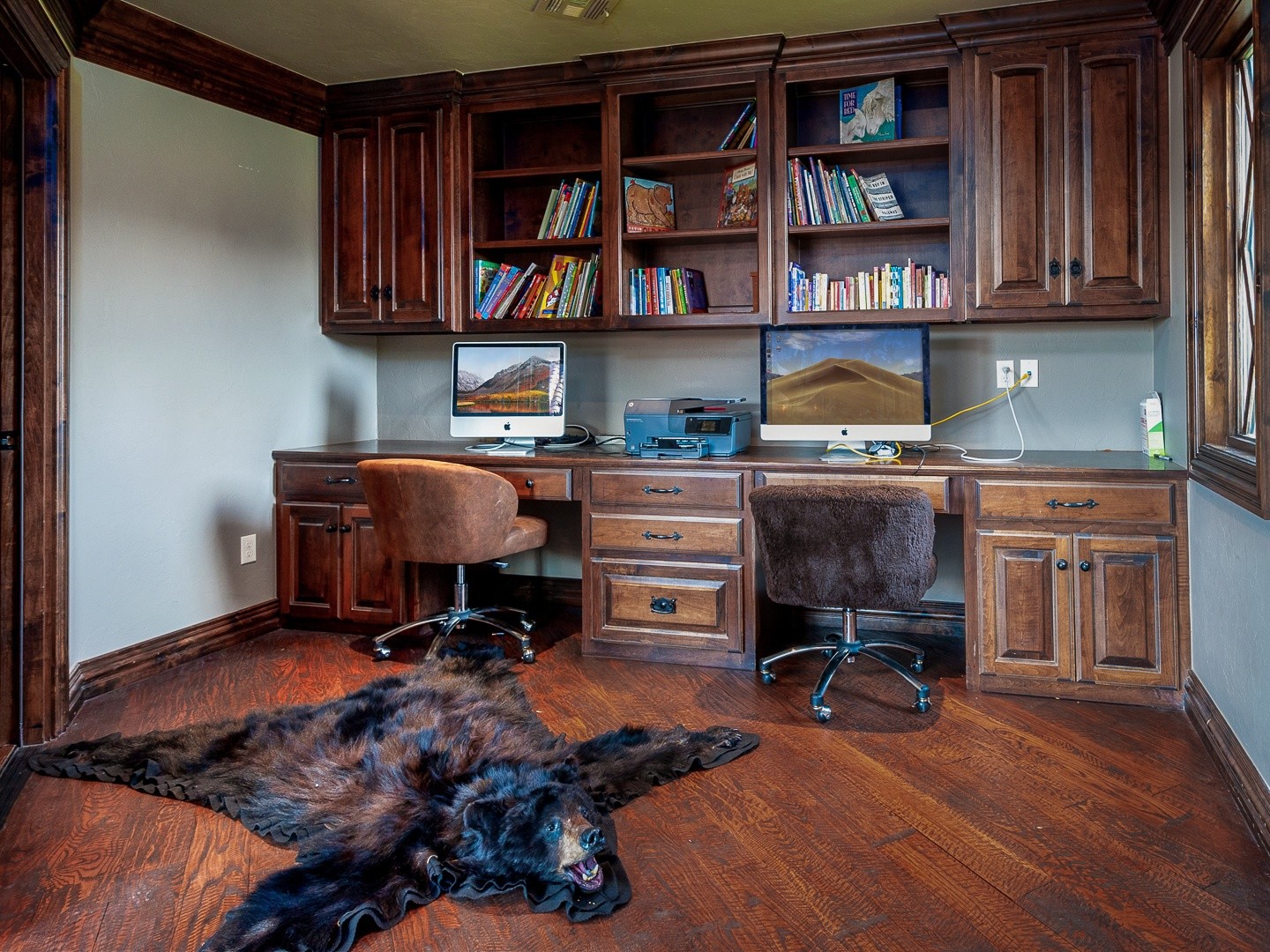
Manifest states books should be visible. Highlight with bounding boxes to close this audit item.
[472,76,952,320]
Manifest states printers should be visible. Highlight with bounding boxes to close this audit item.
[624,396,751,459]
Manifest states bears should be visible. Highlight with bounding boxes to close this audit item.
[7,624,761,952]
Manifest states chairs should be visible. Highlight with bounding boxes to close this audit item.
[748,484,941,723]
[351,456,551,660]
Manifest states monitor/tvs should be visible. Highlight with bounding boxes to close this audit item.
[760,323,932,441]
[450,341,565,438]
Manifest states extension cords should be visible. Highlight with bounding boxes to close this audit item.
[594,434,626,444]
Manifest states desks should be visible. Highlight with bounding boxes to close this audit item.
[270,439,1193,710]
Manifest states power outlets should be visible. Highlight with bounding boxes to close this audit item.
[996,359,1015,388]
[241,533,256,564]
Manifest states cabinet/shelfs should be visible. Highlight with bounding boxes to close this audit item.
[319,1,1172,335]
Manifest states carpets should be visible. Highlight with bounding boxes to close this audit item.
[28,638,761,952]
